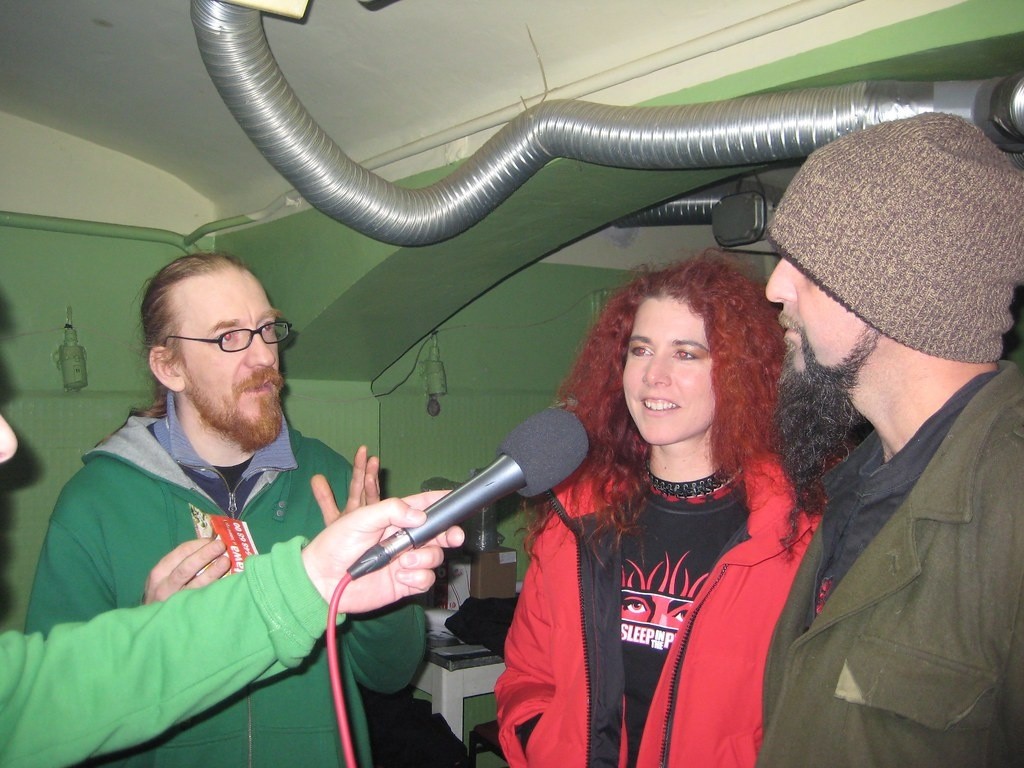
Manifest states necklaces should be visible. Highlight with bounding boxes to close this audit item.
[645,446,736,498]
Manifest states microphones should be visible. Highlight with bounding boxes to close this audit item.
[345,409,591,579]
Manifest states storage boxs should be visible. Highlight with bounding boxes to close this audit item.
[415,547,517,610]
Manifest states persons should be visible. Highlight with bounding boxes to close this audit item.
[755,114,1024,768]
[25,252,426,768]
[493,251,838,768]
[0,415,465,768]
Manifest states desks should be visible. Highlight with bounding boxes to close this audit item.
[409,645,510,768]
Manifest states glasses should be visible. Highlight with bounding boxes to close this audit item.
[162,317,293,352]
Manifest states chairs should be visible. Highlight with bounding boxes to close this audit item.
[469,720,508,768]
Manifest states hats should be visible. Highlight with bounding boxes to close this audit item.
[766,111,1024,359]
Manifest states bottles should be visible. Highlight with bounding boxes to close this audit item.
[467,469,497,551]
[61,331,88,389]
